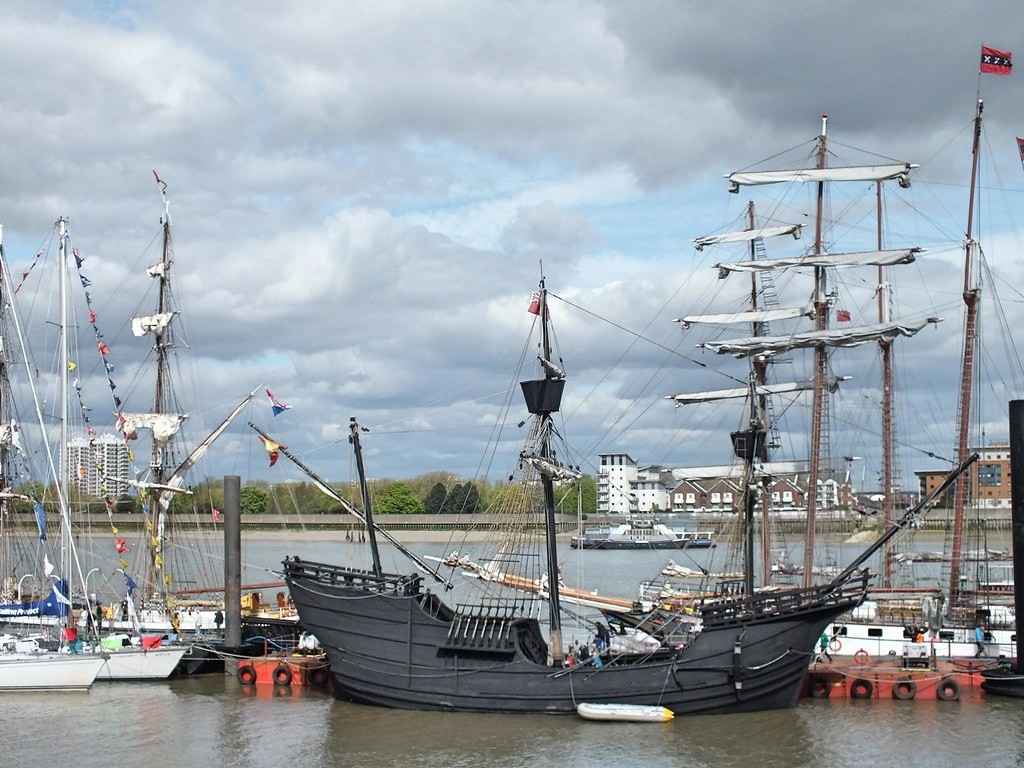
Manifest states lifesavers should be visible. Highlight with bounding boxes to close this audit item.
[851,678,873,699]
[237,665,256,685]
[854,650,869,665]
[937,678,961,701]
[308,667,329,686]
[809,676,831,699]
[829,640,842,651]
[894,678,916,700]
[273,666,292,686]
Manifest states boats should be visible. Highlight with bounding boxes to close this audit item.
[571,517,716,548]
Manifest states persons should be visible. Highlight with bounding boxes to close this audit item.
[194,610,203,640]
[562,621,610,669]
[974,625,985,659]
[214,606,223,637]
[820,633,833,665]
[63,624,77,656]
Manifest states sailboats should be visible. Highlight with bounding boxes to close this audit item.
[0,218,225,680]
[247,261,980,720]
[807,97,1023,699]
[0,169,110,693]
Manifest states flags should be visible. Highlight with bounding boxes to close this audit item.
[980,44,1012,75]
[5,234,288,625]
[836,310,850,322]
[527,289,551,322]
[1017,136,1024,163]
